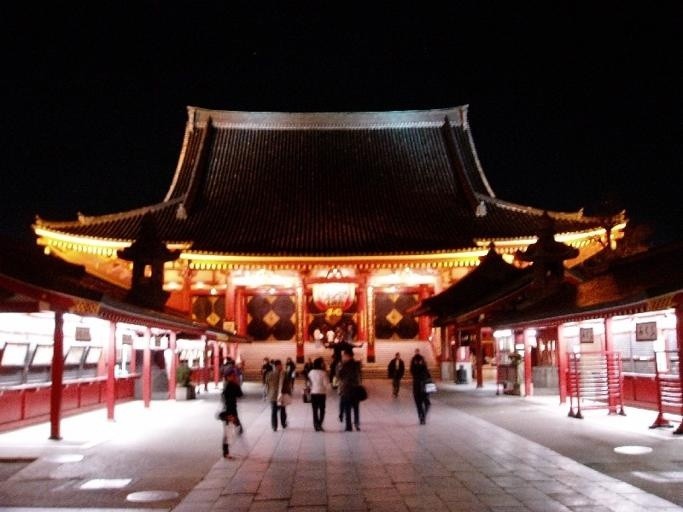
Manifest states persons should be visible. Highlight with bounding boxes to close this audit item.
[217,365,249,460]
[179,361,193,386]
[219,353,345,394]
[409,348,426,378]
[334,349,347,423]
[454,364,466,384]
[218,410,240,460]
[176,359,196,400]
[387,351,405,397]
[307,356,331,432]
[263,360,290,431]
[412,356,433,424]
[337,349,364,431]
[307,320,363,354]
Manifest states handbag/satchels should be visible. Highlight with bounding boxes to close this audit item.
[303,393,311,403]
[425,383,438,393]
[278,394,291,407]
[354,383,367,402]
